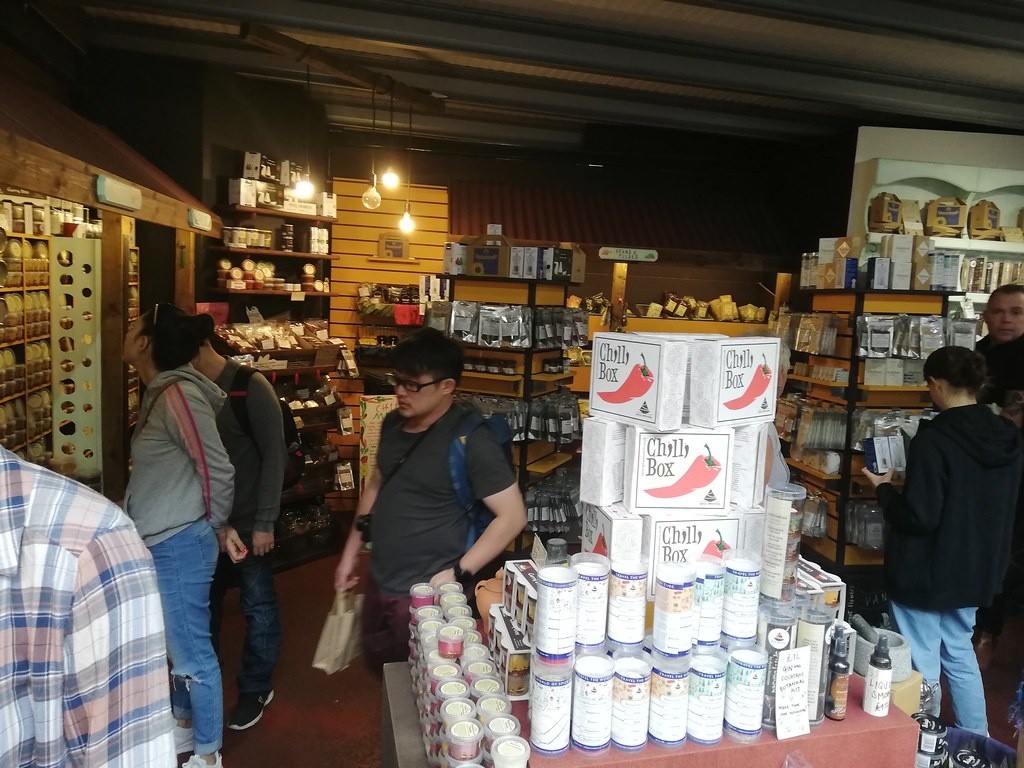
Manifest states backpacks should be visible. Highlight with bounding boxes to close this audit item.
[449,413,513,579]
[230,367,305,491]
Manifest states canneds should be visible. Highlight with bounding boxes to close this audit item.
[222,226,273,251]
[281,223,293,252]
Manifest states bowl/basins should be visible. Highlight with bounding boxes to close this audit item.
[0,198,53,465]
[216,260,325,292]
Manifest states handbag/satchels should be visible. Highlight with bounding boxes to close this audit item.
[312,578,368,676]
[355,515,373,542]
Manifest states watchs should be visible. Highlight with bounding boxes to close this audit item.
[453,563,471,585]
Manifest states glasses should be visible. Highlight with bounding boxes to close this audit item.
[384,371,443,392]
[154,303,171,325]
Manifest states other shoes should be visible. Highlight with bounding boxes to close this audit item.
[974,632,994,670]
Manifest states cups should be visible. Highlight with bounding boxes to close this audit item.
[547,538,568,563]
[408,569,537,768]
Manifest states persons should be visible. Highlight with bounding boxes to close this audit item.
[120,304,301,768]
[0,443,177,768]
[860,344,1024,741]
[973,284,1024,429]
[334,325,529,674]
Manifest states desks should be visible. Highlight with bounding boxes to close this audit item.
[379,621,920,768]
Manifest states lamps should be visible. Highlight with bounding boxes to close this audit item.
[380,79,401,189]
[400,98,415,233]
[294,67,314,198]
[363,85,380,210]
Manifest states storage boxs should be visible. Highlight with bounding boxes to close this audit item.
[926,197,967,227]
[871,192,903,223]
[419,223,572,301]
[666,294,688,316]
[867,235,960,293]
[970,199,1001,229]
[581,332,782,569]
[229,151,337,218]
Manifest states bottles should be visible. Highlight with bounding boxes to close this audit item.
[543,358,563,374]
[455,258,463,274]
[822,625,893,721]
[553,261,563,274]
[83,207,103,239]
[50,203,87,239]
[526,252,535,275]
[862,437,878,473]
[768,300,791,343]
[513,253,521,275]
[800,252,819,289]
[462,357,517,376]
[223,226,273,251]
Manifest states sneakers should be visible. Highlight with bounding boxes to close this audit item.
[182,750,223,768]
[171,715,194,754]
[227,687,275,729]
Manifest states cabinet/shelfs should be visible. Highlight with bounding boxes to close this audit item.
[0,232,140,460]
[776,273,966,623]
[356,305,432,381]
[218,206,339,371]
[442,273,585,558]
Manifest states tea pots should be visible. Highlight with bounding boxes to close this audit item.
[474,567,505,633]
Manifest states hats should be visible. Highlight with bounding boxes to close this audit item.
[174,308,214,339]
